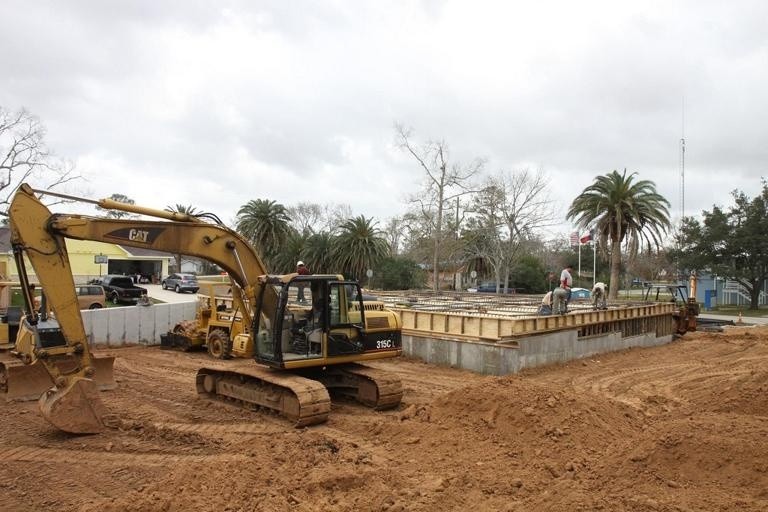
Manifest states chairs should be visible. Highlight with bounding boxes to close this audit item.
[299,299,332,353]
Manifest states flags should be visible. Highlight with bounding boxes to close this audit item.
[571,231,579,246]
[581,229,593,244]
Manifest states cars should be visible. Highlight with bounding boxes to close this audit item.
[161,271,200,294]
[414,260,459,273]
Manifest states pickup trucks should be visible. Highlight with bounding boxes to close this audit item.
[98,274,148,306]
[476,281,516,294]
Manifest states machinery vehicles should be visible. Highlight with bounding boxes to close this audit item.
[159,275,256,357]
[8,173,407,443]
[638,271,704,337]
[0,260,118,402]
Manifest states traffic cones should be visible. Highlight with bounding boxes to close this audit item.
[735,310,744,323]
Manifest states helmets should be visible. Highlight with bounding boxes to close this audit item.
[297,260,305,267]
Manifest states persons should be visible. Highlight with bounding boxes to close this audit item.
[590,282,609,311]
[538,265,573,316]
[294,259,309,302]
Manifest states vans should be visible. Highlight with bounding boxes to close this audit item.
[30,283,106,314]
